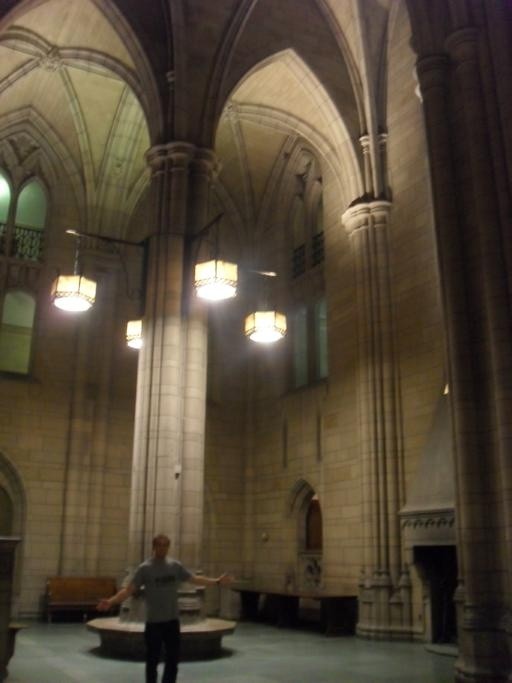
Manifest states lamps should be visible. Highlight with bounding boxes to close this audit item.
[49,211,289,350]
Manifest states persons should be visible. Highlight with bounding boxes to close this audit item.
[96,534,236,683]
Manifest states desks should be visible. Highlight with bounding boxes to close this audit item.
[230,583,359,638]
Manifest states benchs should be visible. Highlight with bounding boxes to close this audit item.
[44,577,120,624]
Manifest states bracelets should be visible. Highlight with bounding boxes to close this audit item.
[217,577,219,584]
